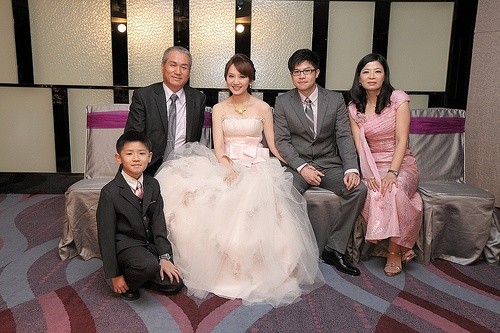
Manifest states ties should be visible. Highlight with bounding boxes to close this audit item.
[136,181,143,199]
[163,94,179,164]
[304,97,315,139]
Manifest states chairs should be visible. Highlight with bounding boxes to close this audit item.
[58,103,131,263]
[275,93,363,264]
[199,107,212,150]
[408,109,500,266]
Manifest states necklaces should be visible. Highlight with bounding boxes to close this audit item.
[231,93,251,116]
[366,100,376,107]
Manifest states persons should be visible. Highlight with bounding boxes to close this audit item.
[95,130,184,300]
[152,52,326,308]
[346,53,424,276]
[273,48,368,276]
[123,46,212,178]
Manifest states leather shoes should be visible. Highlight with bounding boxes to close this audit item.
[322,246,361,276]
[121,286,139,300]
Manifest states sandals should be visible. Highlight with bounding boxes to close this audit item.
[384,249,417,276]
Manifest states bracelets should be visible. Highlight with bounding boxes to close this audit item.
[388,169,399,178]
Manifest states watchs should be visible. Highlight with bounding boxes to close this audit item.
[159,253,171,260]
